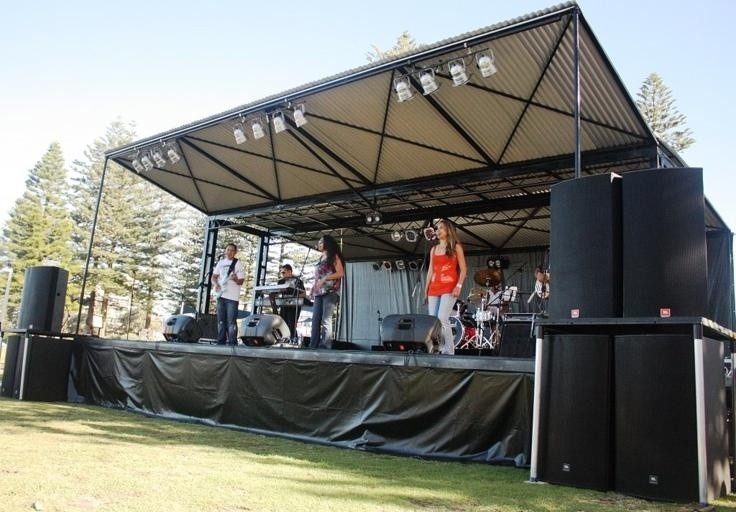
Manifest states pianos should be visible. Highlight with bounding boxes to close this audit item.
[254,297,313,307]
[254,283,304,295]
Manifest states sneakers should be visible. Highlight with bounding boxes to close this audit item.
[439,346,455,356]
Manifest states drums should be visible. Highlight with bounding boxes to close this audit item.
[467,287,486,304]
[475,310,492,322]
[449,315,478,348]
[452,299,469,315]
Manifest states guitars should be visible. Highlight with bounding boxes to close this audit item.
[212,268,240,299]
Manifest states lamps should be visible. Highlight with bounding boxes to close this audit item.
[231,98,308,145]
[391,49,498,104]
[128,141,181,175]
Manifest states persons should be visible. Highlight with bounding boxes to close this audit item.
[486,283,506,336]
[277,264,307,346]
[210,242,245,348]
[534,268,551,313]
[421,219,467,357]
[308,235,346,352]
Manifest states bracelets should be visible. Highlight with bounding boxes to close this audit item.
[456,283,463,288]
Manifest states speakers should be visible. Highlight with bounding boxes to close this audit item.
[16,266,68,333]
[0,336,73,402]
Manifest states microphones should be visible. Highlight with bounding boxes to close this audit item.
[310,244,317,249]
[217,252,227,258]
[430,231,440,241]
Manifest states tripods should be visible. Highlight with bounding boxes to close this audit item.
[459,280,502,349]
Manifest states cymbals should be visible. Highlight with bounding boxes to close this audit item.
[474,269,502,287]
